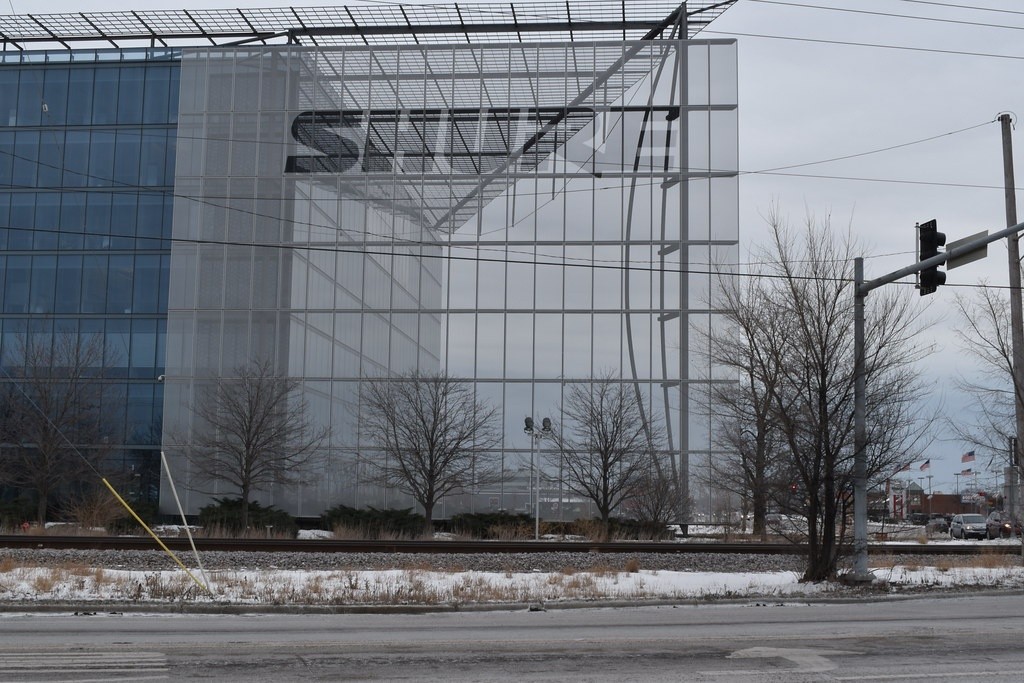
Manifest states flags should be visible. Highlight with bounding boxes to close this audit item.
[920,459,930,471]
[961,451,975,463]
[961,469,971,476]
[893,463,910,474]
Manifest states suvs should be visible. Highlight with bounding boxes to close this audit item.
[949,513,988,540]
[986,510,1024,540]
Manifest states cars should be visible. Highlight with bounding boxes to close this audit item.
[699,512,823,525]
[912,512,954,526]
[926,520,949,535]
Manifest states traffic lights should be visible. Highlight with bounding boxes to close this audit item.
[792,484,797,493]
[919,219,947,297]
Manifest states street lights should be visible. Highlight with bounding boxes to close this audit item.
[926,475,933,521]
[907,479,913,519]
[524,417,552,540]
[972,472,981,512]
[991,469,1002,510]
[918,477,924,513]
[954,473,962,514]
